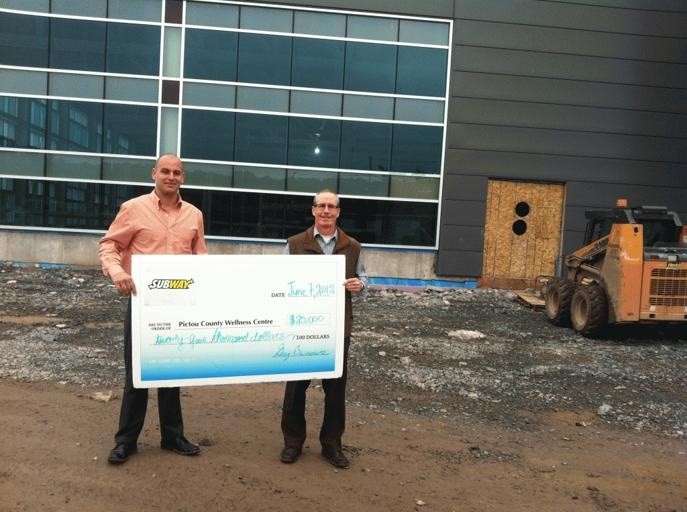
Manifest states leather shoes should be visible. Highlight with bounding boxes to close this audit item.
[108,445,137,463]
[160,438,201,455]
[281,445,301,462]
[322,446,349,468]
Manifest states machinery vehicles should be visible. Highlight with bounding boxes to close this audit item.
[517,194,686,343]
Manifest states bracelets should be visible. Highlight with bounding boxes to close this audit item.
[361,282,364,290]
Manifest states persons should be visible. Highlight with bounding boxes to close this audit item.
[96,153,207,461]
[279,188,367,467]
